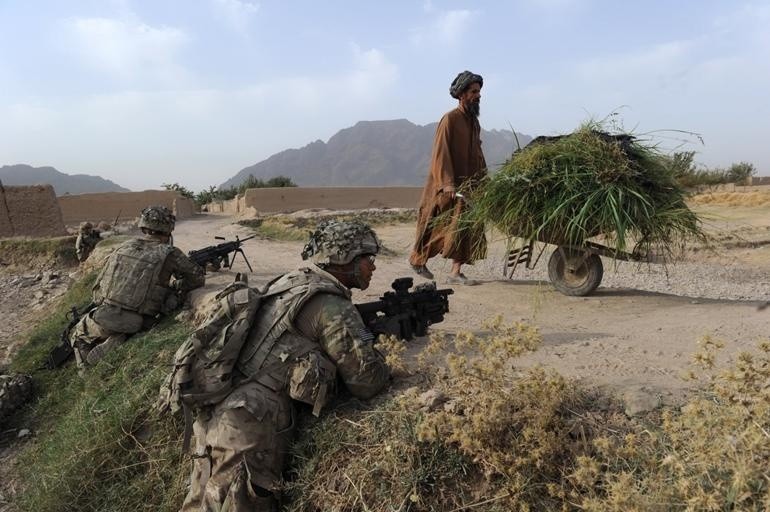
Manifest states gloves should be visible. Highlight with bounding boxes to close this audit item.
[414,281,436,293]
[372,312,409,341]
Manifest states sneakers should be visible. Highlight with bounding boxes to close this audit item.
[446,272,476,287]
[410,259,434,279]
[86,331,127,365]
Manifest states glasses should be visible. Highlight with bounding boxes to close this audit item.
[365,255,376,264]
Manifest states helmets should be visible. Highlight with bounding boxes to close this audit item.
[80,221,92,231]
[303,221,381,266]
[137,205,175,234]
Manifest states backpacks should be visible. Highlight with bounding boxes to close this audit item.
[0,371,33,418]
[153,272,335,453]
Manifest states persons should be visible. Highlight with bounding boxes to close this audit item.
[74,221,103,262]
[172,217,401,512]
[68,205,206,380]
[408,70,488,286]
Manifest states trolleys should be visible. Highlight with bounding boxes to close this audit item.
[455,190,695,298]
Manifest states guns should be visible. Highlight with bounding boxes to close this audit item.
[188,237,255,272]
[354,277,454,341]
